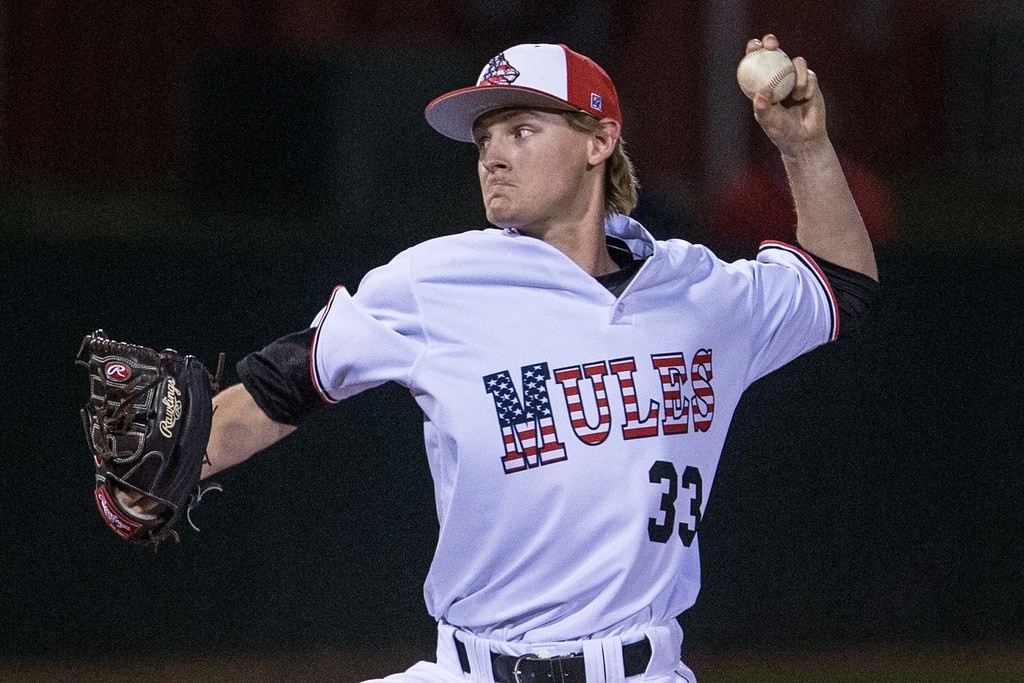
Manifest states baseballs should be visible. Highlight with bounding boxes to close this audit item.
[736,47,798,104]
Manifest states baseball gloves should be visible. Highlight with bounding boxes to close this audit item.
[76,327,214,548]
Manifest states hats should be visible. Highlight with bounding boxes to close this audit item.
[425,44,622,144]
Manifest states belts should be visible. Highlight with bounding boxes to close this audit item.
[453,630,651,683]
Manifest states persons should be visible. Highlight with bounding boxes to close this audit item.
[73,34,887,683]
